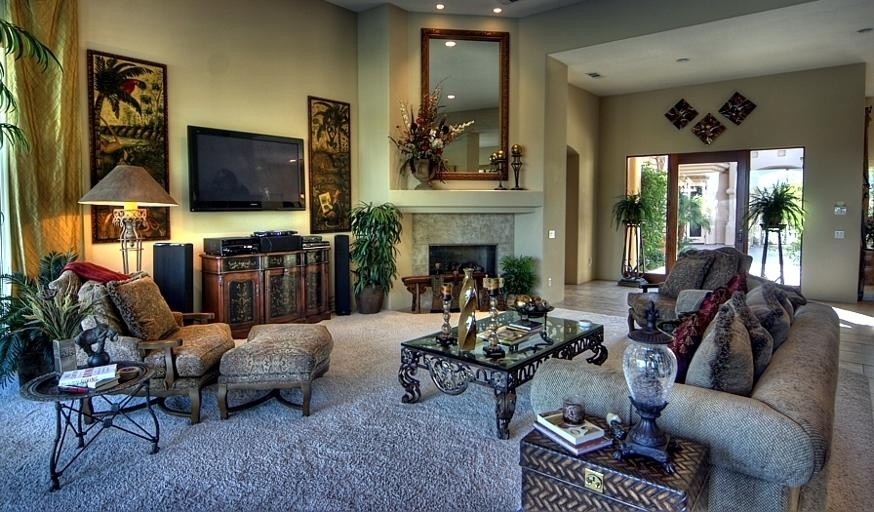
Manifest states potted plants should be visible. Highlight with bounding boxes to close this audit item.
[609,192,654,232]
[737,183,809,241]
[0,247,122,390]
[347,200,404,314]
[499,255,540,311]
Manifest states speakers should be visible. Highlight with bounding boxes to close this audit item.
[153,242,194,325]
[334,235,350,316]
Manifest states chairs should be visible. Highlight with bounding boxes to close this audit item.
[627,248,752,333]
[50,270,234,424]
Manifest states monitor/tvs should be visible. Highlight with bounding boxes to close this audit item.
[187,125,306,212]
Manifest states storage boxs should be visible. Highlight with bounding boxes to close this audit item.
[519,415,711,512]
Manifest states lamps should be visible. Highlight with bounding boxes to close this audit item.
[77,164,180,276]
[612,300,683,473]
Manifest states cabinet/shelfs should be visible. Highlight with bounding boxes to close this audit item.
[199,247,331,340]
[760,224,786,284]
[617,224,648,288]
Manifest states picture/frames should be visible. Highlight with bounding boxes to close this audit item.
[86,48,171,244]
[307,95,352,233]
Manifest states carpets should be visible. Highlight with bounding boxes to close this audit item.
[0,309,873,512]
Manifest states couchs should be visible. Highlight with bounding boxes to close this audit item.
[530,301,840,512]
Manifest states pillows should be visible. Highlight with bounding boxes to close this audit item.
[661,250,794,395]
[106,273,179,342]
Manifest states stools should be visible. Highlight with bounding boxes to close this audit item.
[218,323,333,420]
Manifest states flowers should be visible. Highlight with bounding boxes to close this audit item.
[389,84,474,184]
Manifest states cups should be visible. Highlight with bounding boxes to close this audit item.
[561,394,584,425]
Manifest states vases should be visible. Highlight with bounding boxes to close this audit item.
[410,159,437,190]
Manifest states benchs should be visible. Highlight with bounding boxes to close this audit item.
[402,274,486,312]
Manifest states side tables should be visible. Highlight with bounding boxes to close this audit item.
[20,361,160,492]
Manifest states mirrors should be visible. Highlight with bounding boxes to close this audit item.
[420,28,510,180]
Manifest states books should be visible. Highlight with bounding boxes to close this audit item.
[476,319,544,345]
[533,409,613,456]
[57,364,120,393]
